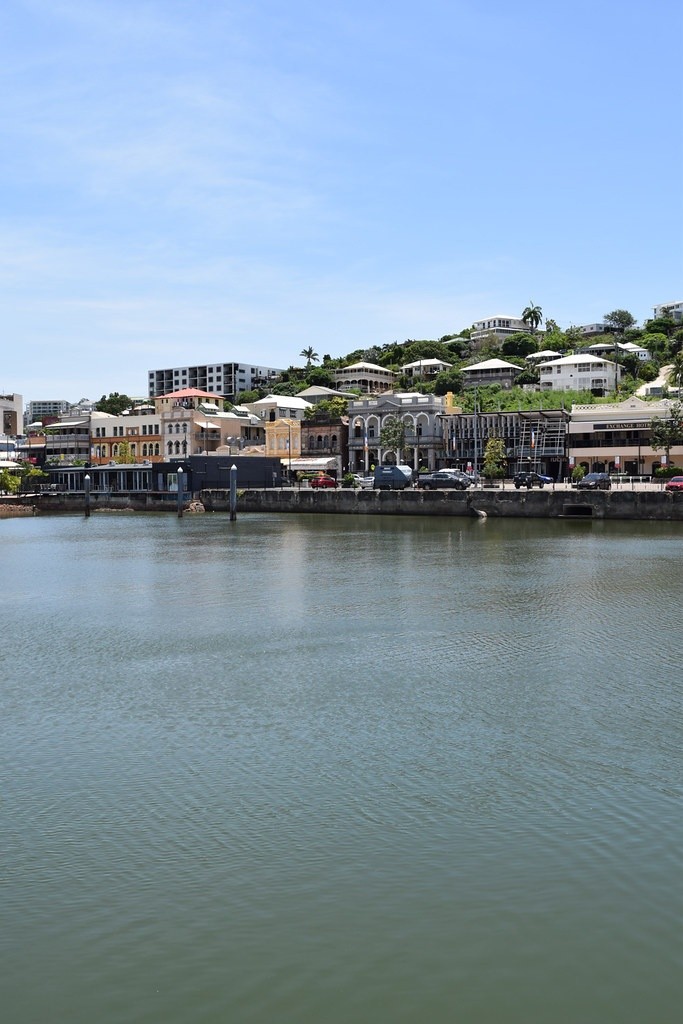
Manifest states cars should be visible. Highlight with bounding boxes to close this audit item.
[513,472,554,489]
[310,477,338,489]
[666,476,683,491]
[360,477,375,489]
[417,468,480,491]
[578,472,611,490]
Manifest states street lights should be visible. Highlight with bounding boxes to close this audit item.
[468,379,482,488]
[283,423,291,488]
[614,331,618,393]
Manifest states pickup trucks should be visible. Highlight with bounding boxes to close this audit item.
[332,474,364,483]
